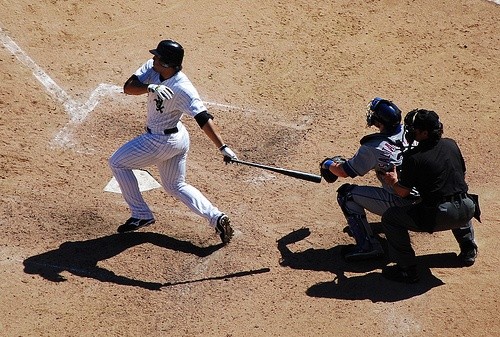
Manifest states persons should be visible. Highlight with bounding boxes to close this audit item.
[381,108,478,281]
[320,98,418,258]
[108,39,240,246]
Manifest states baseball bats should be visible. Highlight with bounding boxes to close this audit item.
[223,155,322,184]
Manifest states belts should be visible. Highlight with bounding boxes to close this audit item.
[146,126,178,135]
[403,195,419,201]
[446,193,465,202]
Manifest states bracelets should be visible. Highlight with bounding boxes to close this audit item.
[219,144,228,151]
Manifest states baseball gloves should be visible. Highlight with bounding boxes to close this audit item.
[319,155,347,184]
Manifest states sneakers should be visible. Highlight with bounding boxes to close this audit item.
[383,263,420,284]
[116,217,156,233]
[457,251,475,266]
[215,214,235,243]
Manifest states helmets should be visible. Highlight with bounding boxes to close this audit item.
[149,40,185,68]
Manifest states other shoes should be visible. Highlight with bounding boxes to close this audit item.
[340,243,384,262]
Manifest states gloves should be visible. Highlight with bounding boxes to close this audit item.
[147,83,175,101]
[219,144,239,165]
